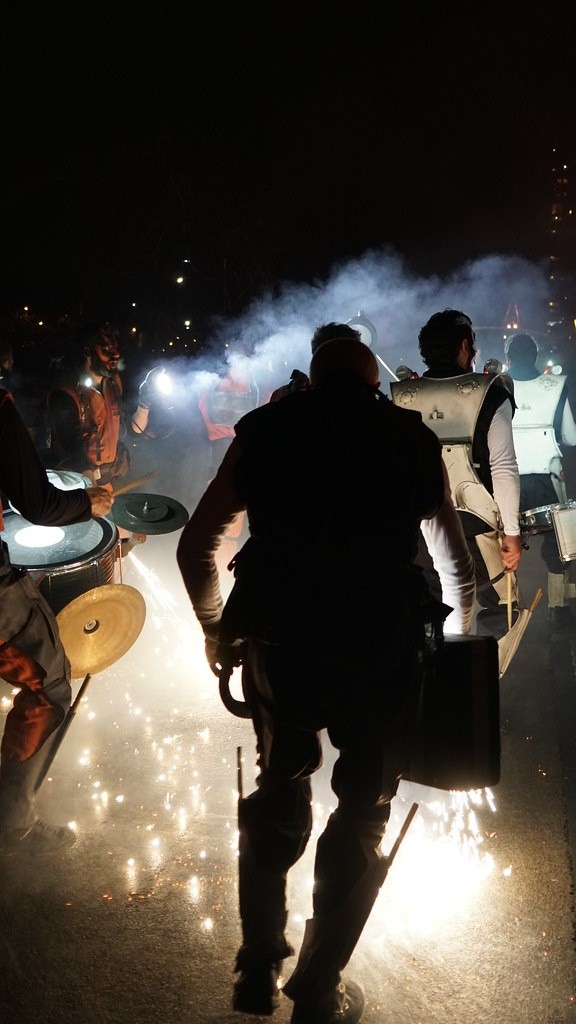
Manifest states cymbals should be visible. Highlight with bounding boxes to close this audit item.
[52,582,148,682]
[105,491,190,537]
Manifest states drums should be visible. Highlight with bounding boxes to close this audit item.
[522,500,576,537]
[0,507,120,616]
[43,466,94,492]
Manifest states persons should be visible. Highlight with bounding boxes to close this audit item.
[176,322,477,1024]
[0,302,576,856]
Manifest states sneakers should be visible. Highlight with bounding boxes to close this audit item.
[0,820,75,856]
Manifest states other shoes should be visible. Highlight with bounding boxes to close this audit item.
[289,981,364,1024]
[232,964,282,1015]
[551,606,576,627]
